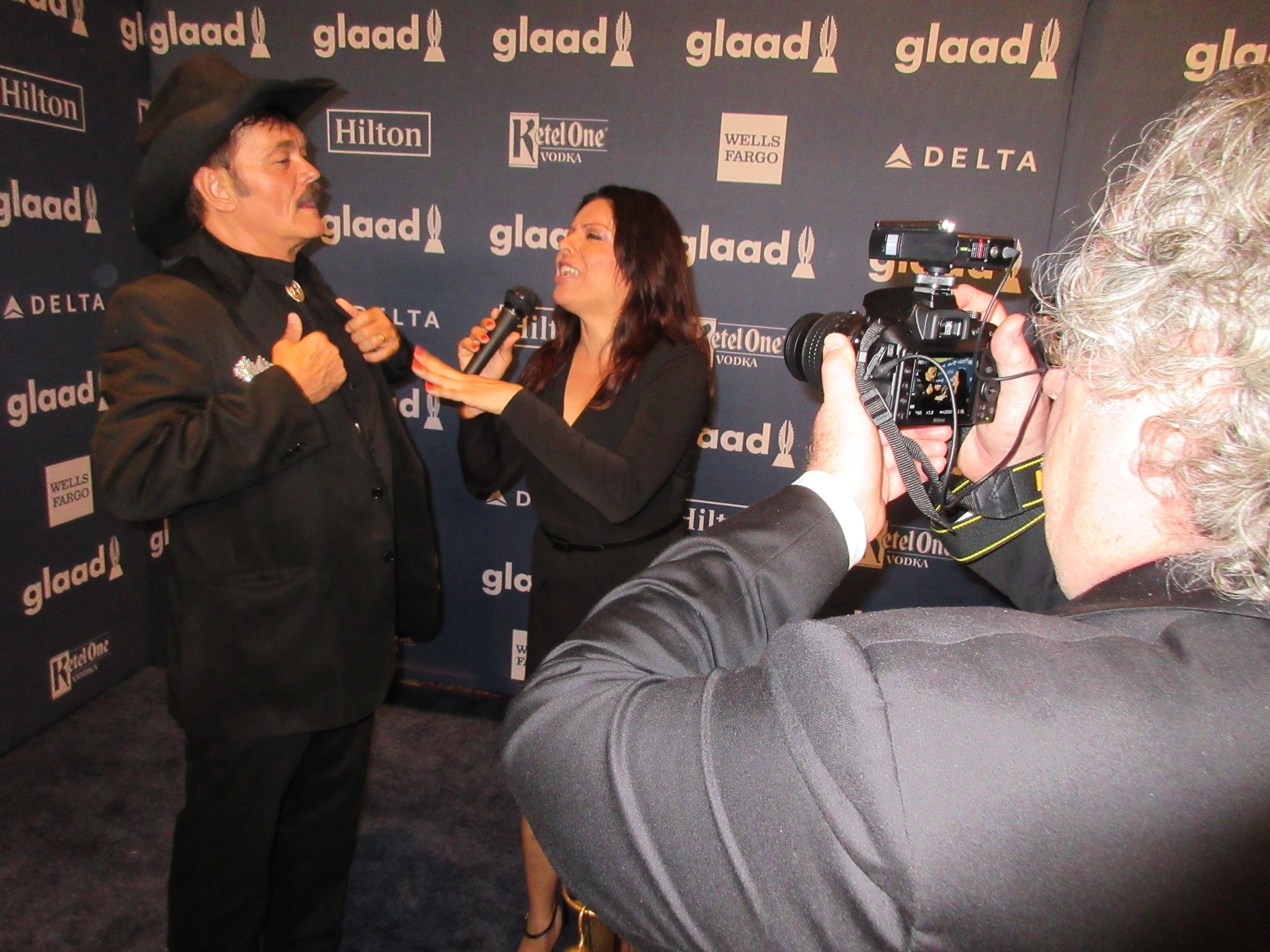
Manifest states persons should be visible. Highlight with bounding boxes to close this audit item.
[496,60,1270,952]
[87,49,451,952]
[410,180,719,952]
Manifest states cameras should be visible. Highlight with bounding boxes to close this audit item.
[782,220,1022,425]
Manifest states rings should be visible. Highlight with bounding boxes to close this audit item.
[378,334,386,345]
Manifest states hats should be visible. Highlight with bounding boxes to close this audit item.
[126,49,337,260]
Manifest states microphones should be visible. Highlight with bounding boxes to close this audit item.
[461,286,539,376]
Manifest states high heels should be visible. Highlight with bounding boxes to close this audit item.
[523,904,572,952]
